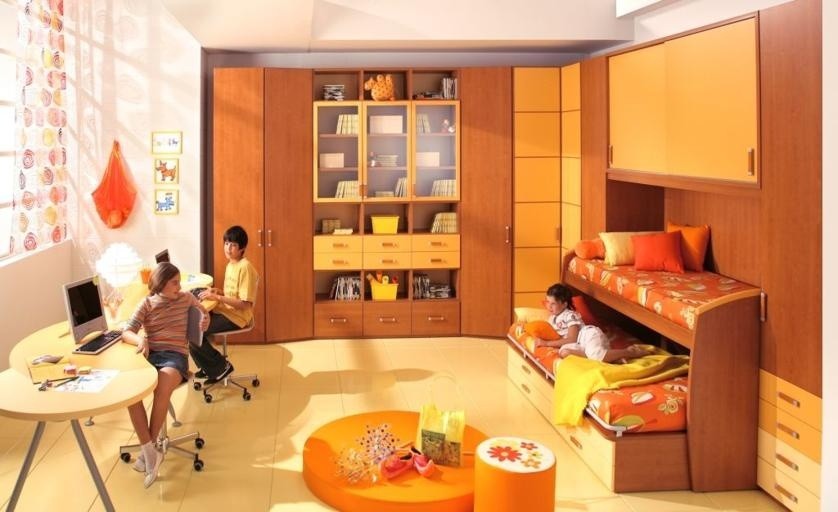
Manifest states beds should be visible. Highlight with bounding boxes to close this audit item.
[507,245,762,495]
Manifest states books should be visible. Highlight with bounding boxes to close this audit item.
[26,353,79,385]
[319,74,461,302]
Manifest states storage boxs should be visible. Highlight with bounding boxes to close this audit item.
[413,150,440,169]
[367,114,404,133]
[318,153,349,168]
[371,214,400,234]
[371,280,398,300]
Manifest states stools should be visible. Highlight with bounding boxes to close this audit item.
[473,435,556,511]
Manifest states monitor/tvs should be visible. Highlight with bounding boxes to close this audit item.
[155,248,168,264]
[63,275,107,343]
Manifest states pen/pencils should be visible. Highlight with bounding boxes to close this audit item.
[55,376,79,388]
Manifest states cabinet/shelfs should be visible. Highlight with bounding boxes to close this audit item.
[211,66,315,349]
[603,11,761,199]
[456,65,560,345]
[754,2,822,512]
[560,57,664,269]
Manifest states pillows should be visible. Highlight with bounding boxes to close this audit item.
[576,224,712,274]
[514,295,608,342]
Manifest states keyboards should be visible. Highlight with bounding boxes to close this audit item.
[189,288,209,300]
[72,330,122,354]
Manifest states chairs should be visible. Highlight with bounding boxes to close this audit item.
[192,277,266,405]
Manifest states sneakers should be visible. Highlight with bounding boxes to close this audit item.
[203,361,233,386]
[194,367,210,379]
[380,453,413,478]
[412,446,436,476]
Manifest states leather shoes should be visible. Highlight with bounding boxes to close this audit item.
[134,456,147,473]
[144,452,164,487]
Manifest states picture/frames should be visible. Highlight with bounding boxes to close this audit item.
[153,157,179,186]
[153,187,180,217]
[149,131,184,156]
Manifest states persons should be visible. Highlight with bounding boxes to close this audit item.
[120,262,210,490]
[538,284,658,367]
[190,224,259,387]
